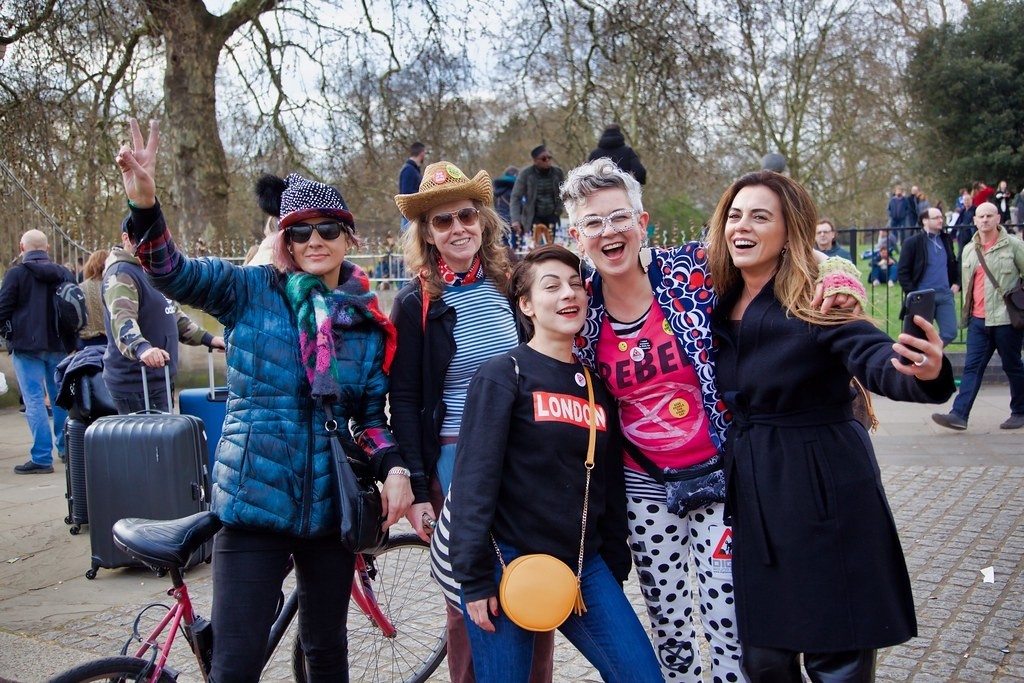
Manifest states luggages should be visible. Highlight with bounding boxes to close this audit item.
[62,346,230,580]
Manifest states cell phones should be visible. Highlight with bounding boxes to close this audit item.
[899,288,937,365]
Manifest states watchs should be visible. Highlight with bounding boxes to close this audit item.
[388,468,411,479]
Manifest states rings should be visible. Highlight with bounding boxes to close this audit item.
[914,354,926,367]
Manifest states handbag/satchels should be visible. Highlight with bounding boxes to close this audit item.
[499,554,587,632]
[1004,277,1024,330]
[329,429,389,554]
[849,376,878,437]
[662,453,728,515]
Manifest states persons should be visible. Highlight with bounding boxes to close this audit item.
[388,161,555,683]
[115,117,414,683]
[390,123,1024,683]
[0,173,287,475]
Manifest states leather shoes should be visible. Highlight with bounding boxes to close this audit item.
[61,456,65,463]
[13,460,54,474]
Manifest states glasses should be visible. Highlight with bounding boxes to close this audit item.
[535,156,552,162]
[814,230,834,237]
[575,209,638,239]
[284,221,343,243]
[927,216,945,221]
[426,207,480,233]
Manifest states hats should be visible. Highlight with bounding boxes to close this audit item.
[254,173,354,231]
[532,145,546,158]
[391,161,493,222]
[122,216,130,232]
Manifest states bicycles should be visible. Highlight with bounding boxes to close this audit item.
[40,509,449,683]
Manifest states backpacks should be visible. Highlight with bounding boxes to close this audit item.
[49,280,88,339]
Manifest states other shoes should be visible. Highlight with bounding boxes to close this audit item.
[873,279,880,287]
[1000,415,1024,429]
[931,412,967,430]
[888,281,894,287]
